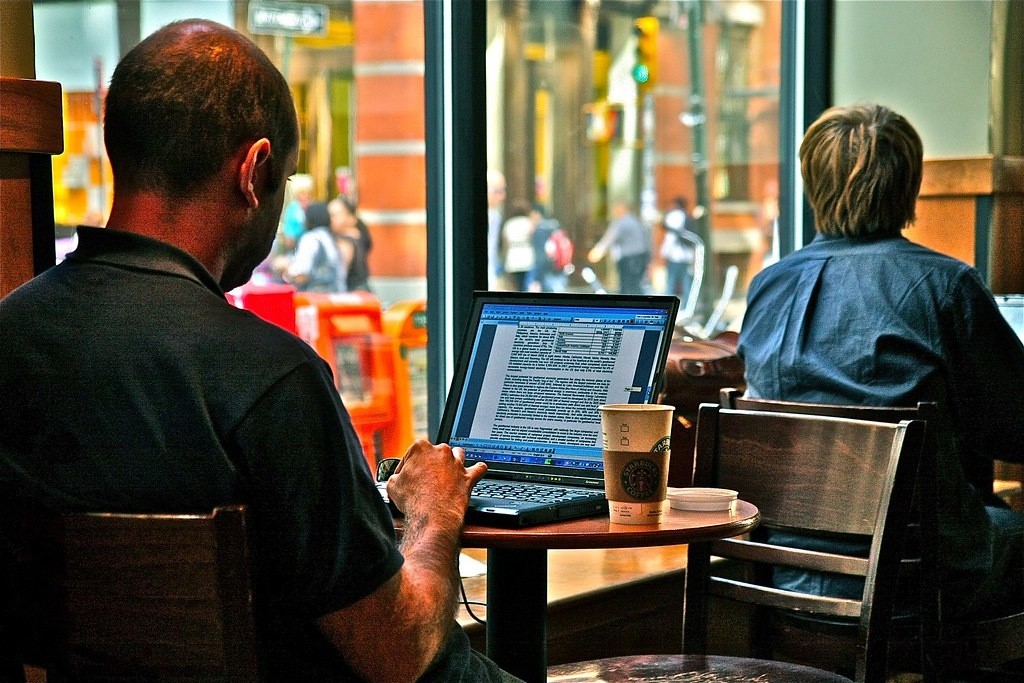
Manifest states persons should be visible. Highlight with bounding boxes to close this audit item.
[660,193,696,311]
[1,17,528,683]
[735,101,1024,683]
[269,173,372,295]
[587,197,651,295]
[500,197,573,293]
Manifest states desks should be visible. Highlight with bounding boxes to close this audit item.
[387,499,760,683]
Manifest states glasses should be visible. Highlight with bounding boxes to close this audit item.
[376,458,401,481]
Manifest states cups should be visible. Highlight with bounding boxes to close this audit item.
[598,403,675,525]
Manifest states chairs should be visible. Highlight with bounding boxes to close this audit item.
[0,502,257,683]
[680,403,929,683]
[718,385,1024,683]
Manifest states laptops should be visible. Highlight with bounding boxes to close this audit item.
[372,292,679,527]
